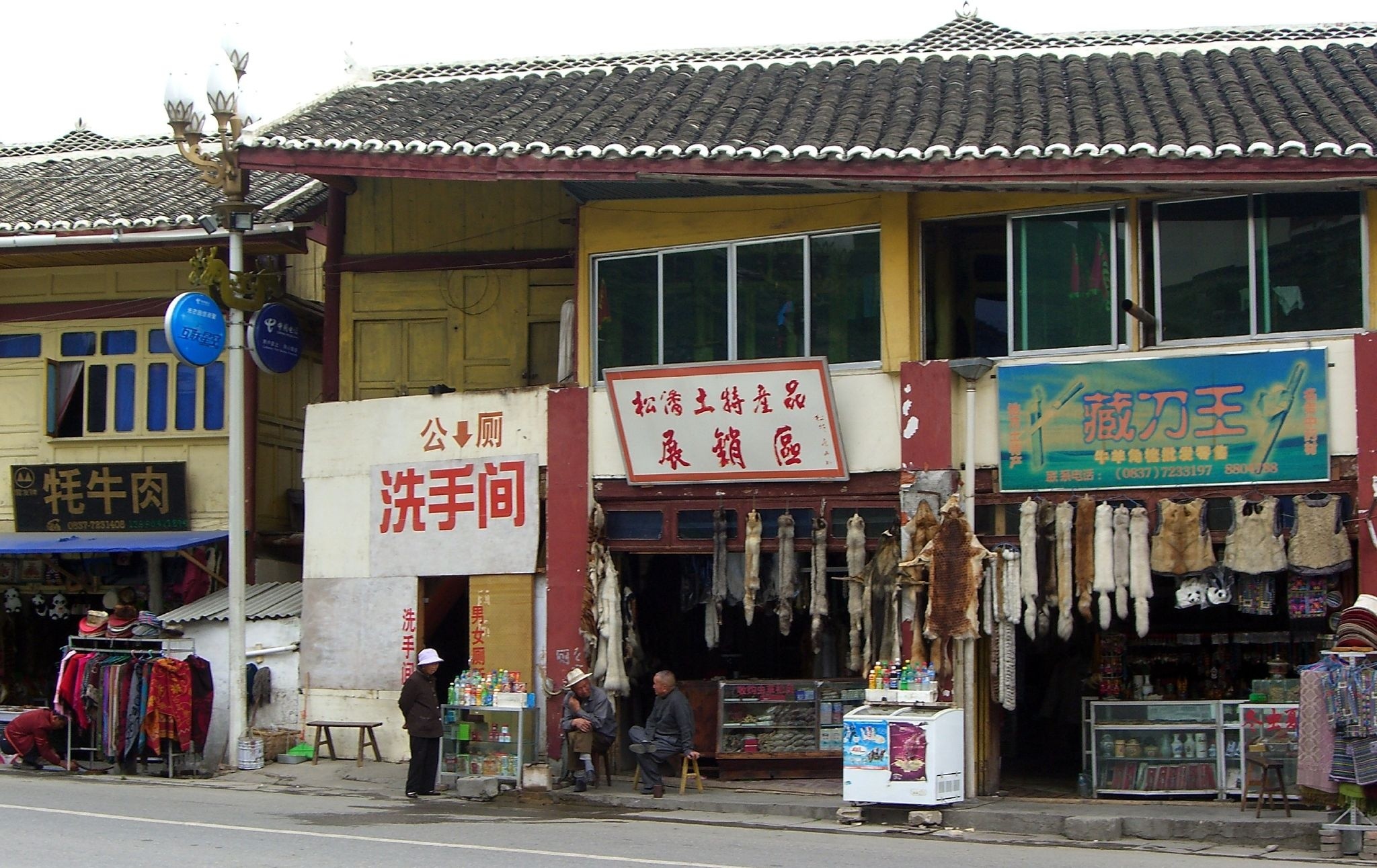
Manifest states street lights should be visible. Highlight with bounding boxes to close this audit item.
[161,27,309,768]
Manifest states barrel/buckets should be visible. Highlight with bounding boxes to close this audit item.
[238,726,265,770]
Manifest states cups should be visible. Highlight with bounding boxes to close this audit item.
[733,672,740,679]
[527,693,535,707]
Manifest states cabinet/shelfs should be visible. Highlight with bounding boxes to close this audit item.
[716,680,869,781]
[437,704,540,787]
[1081,696,1301,800]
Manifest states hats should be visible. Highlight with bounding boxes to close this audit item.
[564,668,593,687]
[76,604,163,639]
[1336,594,1377,649]
[417,649,444,665]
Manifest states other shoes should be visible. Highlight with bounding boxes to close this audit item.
[407,791,419,798]
[12,758,44,770]
[629,742,657,753]
[586,770,596,783]
[420,791,442,797]
[573,779,588,793]
[639,786,666,795]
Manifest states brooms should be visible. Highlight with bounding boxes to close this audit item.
[286,672,314,759]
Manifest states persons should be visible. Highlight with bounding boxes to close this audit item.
[0,708,80,770]
[560,668,618,786]
[629,670,700,798]
[398,648,444,798]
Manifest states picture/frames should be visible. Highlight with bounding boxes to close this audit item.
[0,557,45,583]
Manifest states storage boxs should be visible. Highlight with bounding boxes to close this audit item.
[493,692,536,708]
[0,705,49,721]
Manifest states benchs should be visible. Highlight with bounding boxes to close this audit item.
[633,753,703,795]
[306,721,383,767]
[1241,757,1291,818]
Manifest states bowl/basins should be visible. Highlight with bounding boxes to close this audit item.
[500,671,527,692]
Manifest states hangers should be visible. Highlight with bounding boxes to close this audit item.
[94,647,198,666]
[1169,485,1194,501]
[1302,488,1330,501]
[1295,654,1377,685]
[1018,489,1056,508]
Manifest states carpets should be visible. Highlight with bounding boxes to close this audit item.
[1067,488,1080,509]
[1242,481,1266,503]
[1101,494,1142,510]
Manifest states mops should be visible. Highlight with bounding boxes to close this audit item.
[217,663,258,771]
[250,666,271,726]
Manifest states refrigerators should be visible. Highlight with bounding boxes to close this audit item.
[842,704,966,805]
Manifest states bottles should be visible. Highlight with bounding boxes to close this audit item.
[869,658,935,691]
[446,668,509,706]
[1098,728,1218,759]
[1133,675,1144,699]
[1142,675,1153,695]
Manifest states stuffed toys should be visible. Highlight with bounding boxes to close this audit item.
[1,587,70,621]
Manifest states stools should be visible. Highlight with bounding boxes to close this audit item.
[592,748,612,788]
